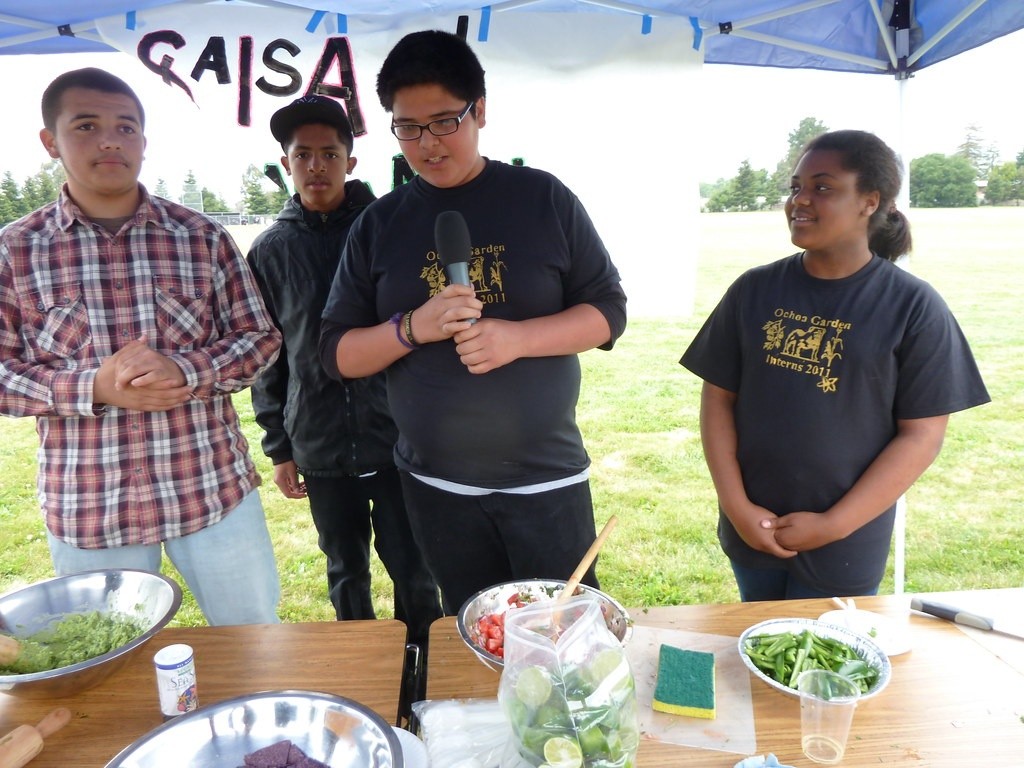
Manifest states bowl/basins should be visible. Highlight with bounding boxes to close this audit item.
[0,569,183,700]
[103,690,403,768]
[456,579,632,674]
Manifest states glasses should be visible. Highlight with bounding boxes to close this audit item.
[391,100,474,141]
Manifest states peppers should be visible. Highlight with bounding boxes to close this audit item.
[745,629,878,699]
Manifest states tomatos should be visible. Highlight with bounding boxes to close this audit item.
[482,594,531,660]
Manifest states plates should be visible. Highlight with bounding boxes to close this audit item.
[822,610,911,655]
[392,727,427,768]
[739,617,891,705]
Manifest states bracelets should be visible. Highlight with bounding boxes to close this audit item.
[405,310,418,345]
[389,312,420,349]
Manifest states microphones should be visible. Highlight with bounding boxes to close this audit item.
[436,210,477,329]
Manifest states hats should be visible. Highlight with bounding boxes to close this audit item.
[270,95,353,147]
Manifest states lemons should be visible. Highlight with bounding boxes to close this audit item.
[508,649,639,768]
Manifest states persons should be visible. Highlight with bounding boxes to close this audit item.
[315,30,628,617]
[678,130,991,603]
[247,95,442,654]
[0,66,282,629]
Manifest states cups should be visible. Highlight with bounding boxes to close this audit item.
[153,644,199,720]
[797,670,861,764]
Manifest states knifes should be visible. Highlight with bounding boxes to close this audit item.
[911,598,1024,637]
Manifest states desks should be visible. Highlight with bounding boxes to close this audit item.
[0,587,1024,767]
